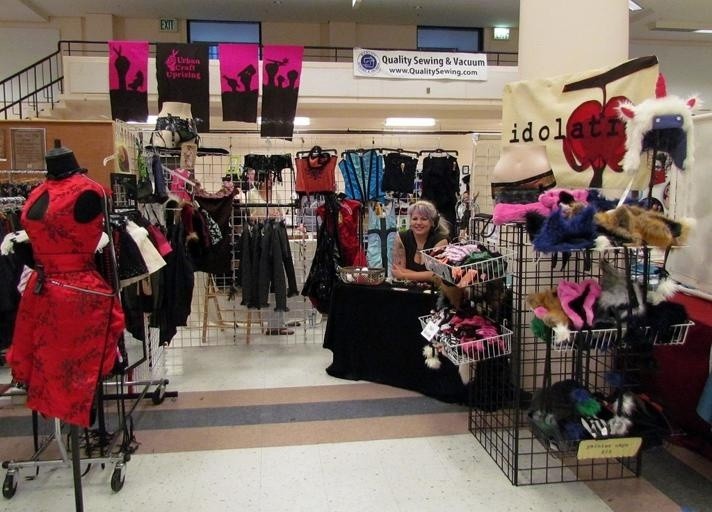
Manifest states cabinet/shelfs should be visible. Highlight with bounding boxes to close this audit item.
[465,213,695,486]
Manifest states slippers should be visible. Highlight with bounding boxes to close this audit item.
[266,327,295,335]
[288,321,300,326]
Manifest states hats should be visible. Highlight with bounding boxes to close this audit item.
[528,267,688,349]
[612,94,704,173]
[493,188,689,247]
[528,377,671,461]
[420,304,506,368]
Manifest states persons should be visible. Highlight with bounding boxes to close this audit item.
[490,79,558,203]
[391,200,449,283]
[7,146,126,428]
[233,171,286,222]
[152,102,198,149]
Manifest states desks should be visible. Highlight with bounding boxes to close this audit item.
[336,279,514,412]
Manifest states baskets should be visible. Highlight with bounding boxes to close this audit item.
[339,266,386,285]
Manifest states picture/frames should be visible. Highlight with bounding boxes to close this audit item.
[10,127,47,172]
